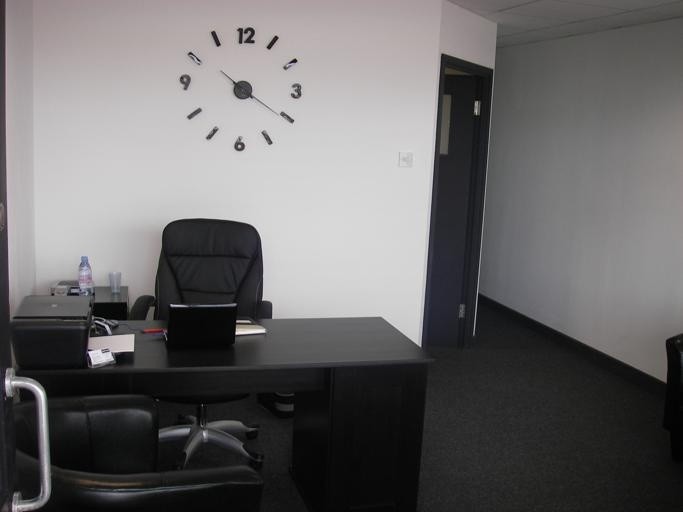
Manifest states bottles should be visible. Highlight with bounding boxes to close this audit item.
[76,256,93,296]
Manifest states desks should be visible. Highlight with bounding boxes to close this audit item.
[16,317,436,512]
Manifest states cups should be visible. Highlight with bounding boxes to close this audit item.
[108,272,121,294]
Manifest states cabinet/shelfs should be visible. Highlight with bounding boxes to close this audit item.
[52,286,128,320]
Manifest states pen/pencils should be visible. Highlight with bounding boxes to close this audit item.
[141,328,164,332]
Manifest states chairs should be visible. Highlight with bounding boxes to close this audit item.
[5,394,267,512]
[128,218,272,469]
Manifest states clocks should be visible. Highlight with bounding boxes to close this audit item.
[179,26,303,151]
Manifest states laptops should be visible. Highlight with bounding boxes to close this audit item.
[163,303,238,353]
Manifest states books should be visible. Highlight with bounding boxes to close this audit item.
[234,315,267,336]
